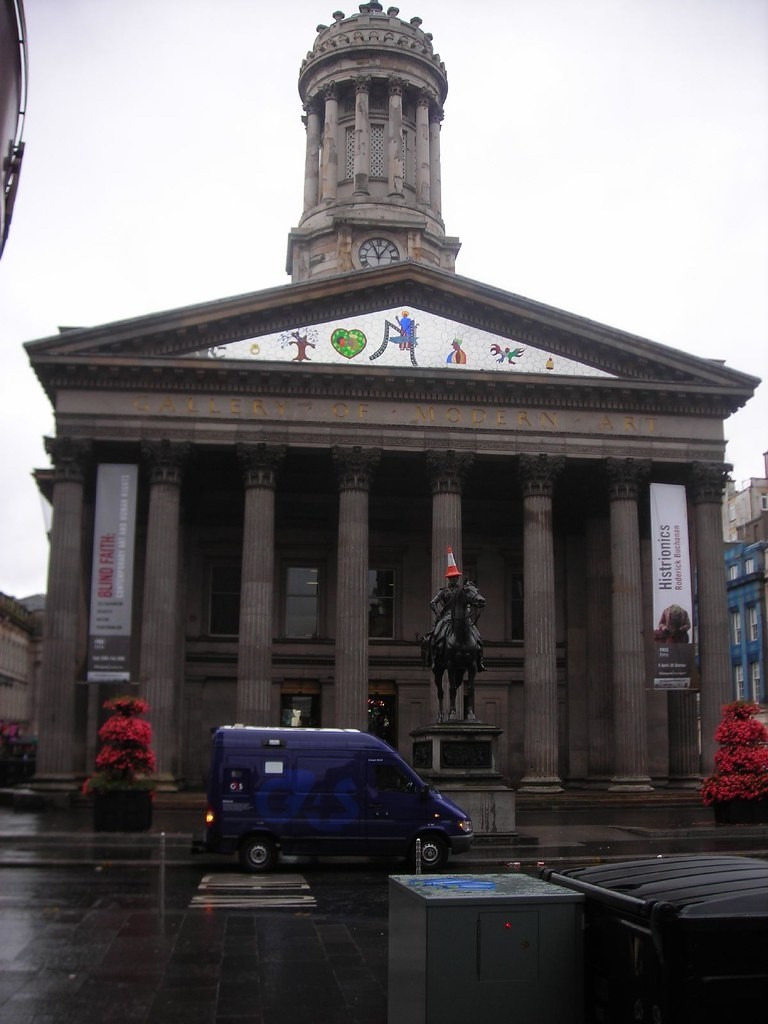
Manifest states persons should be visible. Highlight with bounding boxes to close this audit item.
[429,575,486,671]
[658,605,690,644]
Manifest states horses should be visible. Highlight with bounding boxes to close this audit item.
[415,576,488,724]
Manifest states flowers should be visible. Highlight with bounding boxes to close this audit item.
[696,699,768,807]
[81,694,158,802]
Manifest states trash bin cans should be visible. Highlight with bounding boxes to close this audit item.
[7,737,36,780]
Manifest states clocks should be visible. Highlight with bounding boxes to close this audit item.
[350,230,406,270]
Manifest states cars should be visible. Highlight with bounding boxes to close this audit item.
[1,717,39,789]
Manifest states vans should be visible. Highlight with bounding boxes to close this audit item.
[203,724,476,875]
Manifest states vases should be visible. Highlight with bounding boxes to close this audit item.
[92,790,152,833]
[712,797,768,824]
